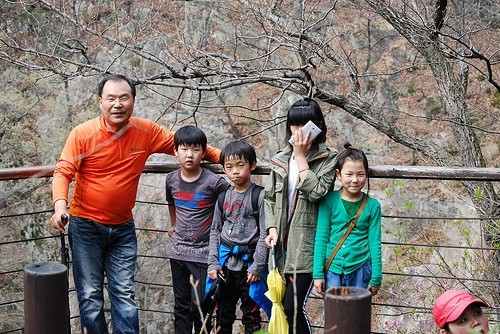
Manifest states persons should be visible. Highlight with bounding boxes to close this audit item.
[48,74,222,334]
[165,125,231,334]
[433,289,489,334]
[207,141,268,334]
[264,98,340,334]
[313,142,382,296]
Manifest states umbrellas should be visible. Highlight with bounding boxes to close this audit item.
[264,240,289,334]
[60,213,69,267]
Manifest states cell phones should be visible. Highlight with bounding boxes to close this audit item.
[287,120,321,146]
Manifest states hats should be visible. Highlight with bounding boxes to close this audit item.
[433,288,487,329]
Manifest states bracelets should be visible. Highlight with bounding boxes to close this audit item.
[299,169,309,173]
